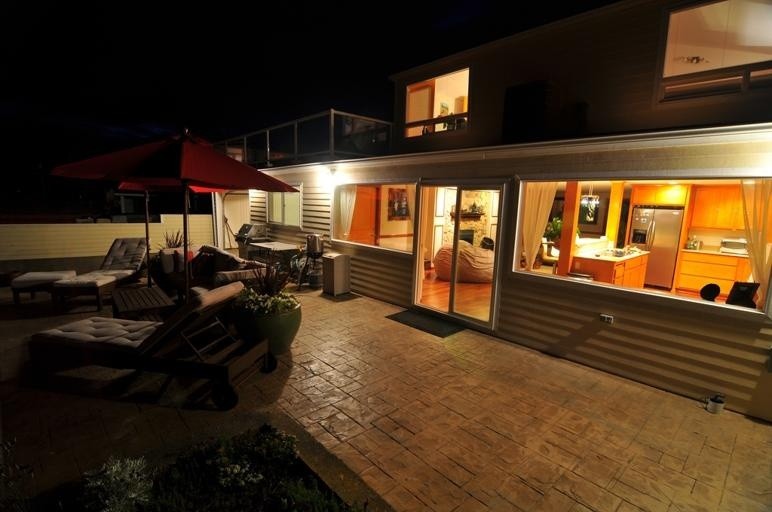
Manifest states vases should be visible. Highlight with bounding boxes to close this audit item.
[233,304,301,357]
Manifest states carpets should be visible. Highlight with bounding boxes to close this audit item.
[385,309,468,339]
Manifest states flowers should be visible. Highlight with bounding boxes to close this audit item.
[232,288,300,314]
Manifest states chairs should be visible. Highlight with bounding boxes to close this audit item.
[51,237,147,311]
[30,282,277,411]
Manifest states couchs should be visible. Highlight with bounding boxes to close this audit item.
[149,244,277,299]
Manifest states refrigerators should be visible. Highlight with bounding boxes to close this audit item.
[628,207,685,289]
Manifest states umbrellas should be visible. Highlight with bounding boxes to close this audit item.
[47,125,300,306]
[119,171,228,289]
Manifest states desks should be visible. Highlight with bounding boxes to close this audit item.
[248,241,301,265]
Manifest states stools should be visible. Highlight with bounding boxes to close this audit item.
[12,269,77,304]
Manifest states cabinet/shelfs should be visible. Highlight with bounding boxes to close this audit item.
[570,254,648,289]
[691,184,771,232]
[676,252,751,301]
